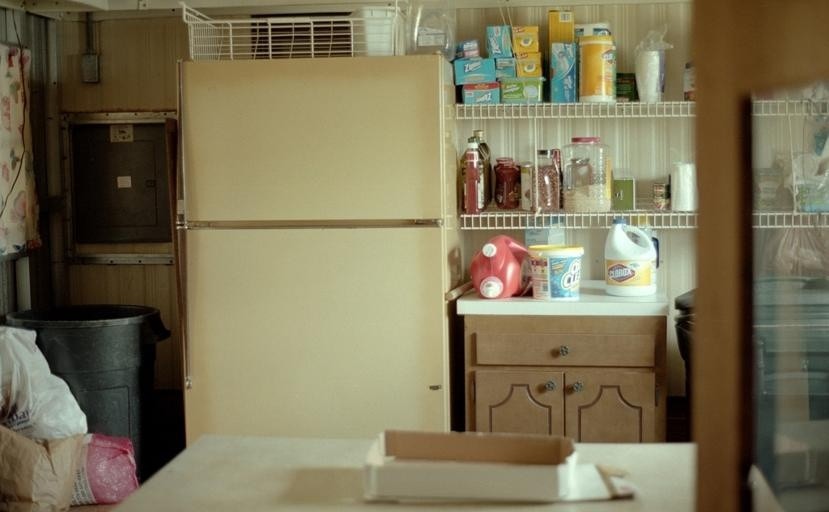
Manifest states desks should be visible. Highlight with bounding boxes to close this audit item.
[106,433,784,511]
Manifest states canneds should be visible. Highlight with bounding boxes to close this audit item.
[649,183,670,212]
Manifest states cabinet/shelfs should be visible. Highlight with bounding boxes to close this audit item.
[453,294,670,442]
[454,97,829,232]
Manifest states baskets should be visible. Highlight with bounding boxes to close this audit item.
[177,2,403,60]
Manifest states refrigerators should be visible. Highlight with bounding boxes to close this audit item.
[177,49,450,442]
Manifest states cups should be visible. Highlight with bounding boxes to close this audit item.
[634,49,665,105]
[669,160,699,212]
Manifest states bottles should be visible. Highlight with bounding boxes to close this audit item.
[457,130,493,214]
[496,148,561,214]
[558,135,613,213]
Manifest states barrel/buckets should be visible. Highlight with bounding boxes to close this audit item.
[604,219,657,298]
[470,236,534,299]
